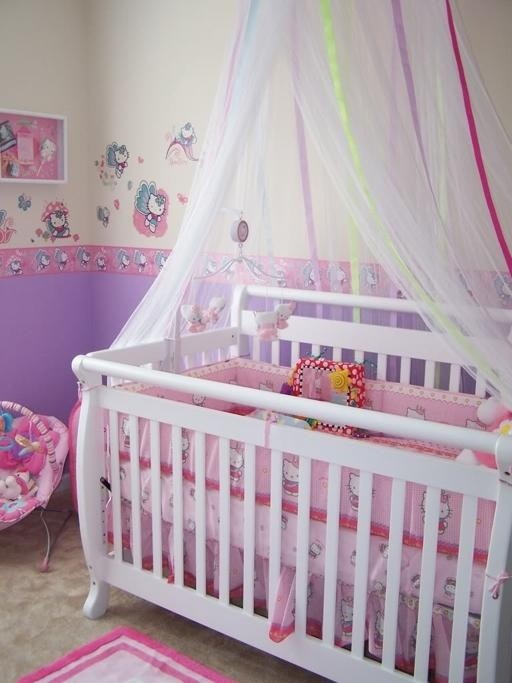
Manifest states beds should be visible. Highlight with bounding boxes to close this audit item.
[69,284,512,683]
[1,400,73,571]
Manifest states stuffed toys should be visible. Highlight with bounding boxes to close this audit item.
[478,397,511,437]
[0,413,59,499]
[256,304,289,338]
[181,297,225,332]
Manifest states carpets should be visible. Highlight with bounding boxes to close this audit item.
[14,627,238,683]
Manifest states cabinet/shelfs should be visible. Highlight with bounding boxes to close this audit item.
[1,109,67,183]
